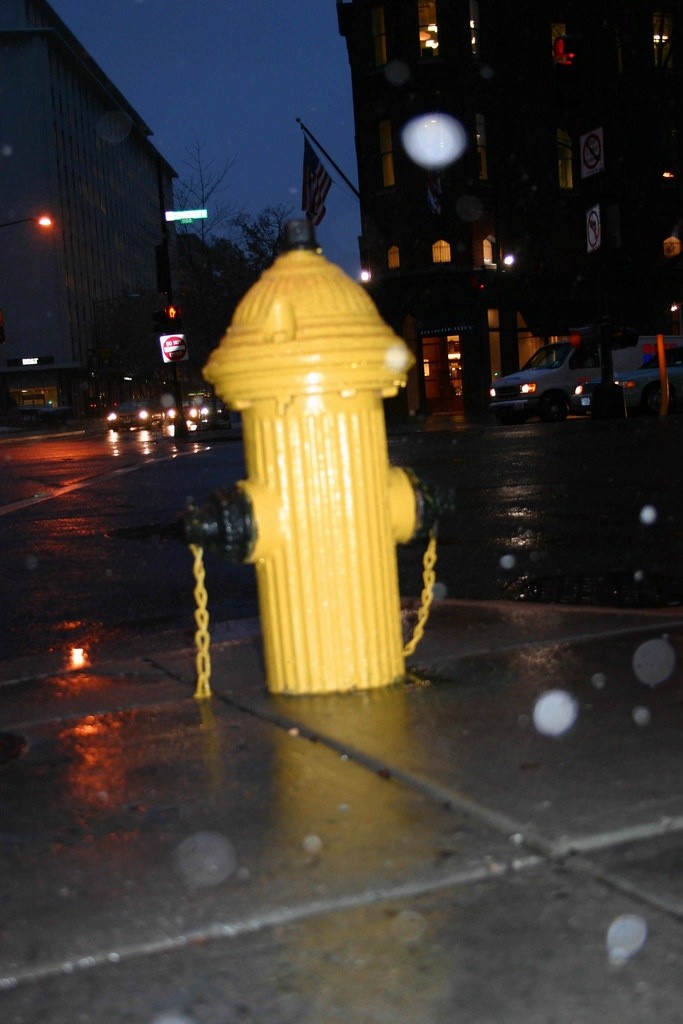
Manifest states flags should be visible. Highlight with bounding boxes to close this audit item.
[295,126,336,230]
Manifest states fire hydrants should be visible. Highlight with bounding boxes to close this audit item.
[201,244,422,695]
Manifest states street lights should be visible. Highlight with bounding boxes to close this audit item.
[92,292,141,379]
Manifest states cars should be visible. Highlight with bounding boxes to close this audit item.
[615,346,683,416]
[103,391,232,436]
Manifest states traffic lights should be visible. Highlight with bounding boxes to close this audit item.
[153,306,181,322]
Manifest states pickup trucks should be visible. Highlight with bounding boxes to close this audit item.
[487,337,622,424]
